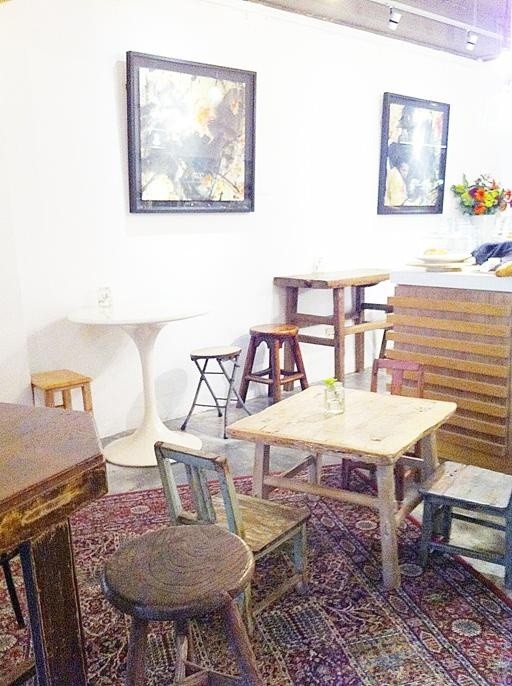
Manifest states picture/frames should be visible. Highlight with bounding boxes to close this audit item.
[127,50,257,212]
[377,92,450,216]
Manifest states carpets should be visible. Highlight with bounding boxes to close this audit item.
[1,464,511,686]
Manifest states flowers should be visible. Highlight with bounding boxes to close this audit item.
[452,174,511,216]
[323,378,343,404]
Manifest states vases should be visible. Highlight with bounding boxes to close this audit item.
[468,210,498,255]
[324,382,345,414]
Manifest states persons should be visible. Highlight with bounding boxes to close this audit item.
[386,105,442,206]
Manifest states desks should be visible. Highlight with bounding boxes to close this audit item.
[0,402,110,684]
[225,385,457,590]
[273,269,390,391]
[68,303,206,467]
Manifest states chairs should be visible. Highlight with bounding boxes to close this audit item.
[342,359,426,504]
[155,441,310,637]
[421,459,511,592]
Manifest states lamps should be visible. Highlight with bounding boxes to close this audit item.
[386,7,479,51]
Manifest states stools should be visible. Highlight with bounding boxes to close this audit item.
[32,369,94,415]
[102,526,263,684]
[180,346,252,440]
[236,325,309,410]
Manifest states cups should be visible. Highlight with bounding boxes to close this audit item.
[95,286,113,308]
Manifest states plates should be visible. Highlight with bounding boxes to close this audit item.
[404,261,463,270]
[414,252,472,262]
